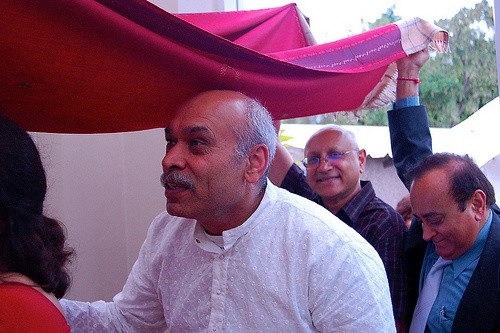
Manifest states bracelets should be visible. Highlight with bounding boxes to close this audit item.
[396,77,420,84]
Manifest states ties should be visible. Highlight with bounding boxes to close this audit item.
[408,256,453,333]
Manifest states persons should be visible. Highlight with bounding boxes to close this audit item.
[395,193,413,229]
[0,111,74,333]
[265,117,408,333]
[55,89,400,333]
[387,45,500,333]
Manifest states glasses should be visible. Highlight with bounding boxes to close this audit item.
[302,149,357,169]
